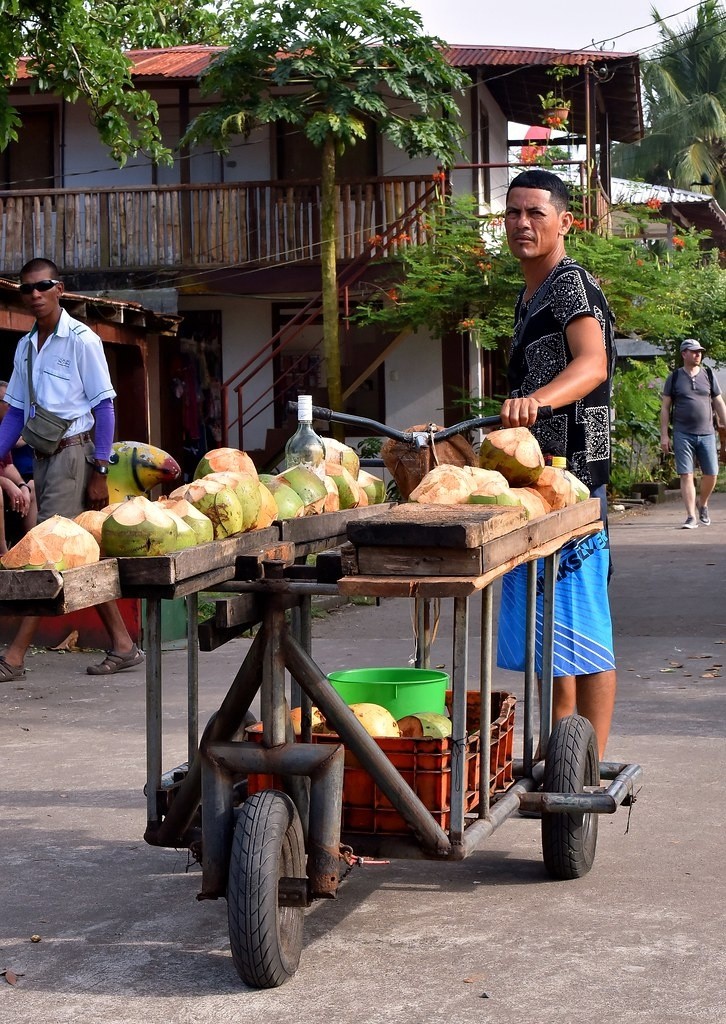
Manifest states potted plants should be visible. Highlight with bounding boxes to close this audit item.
[540,64,571,129]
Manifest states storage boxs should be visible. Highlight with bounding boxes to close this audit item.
[244,688,517,846]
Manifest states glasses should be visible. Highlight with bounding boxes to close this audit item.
[19,279,59,294]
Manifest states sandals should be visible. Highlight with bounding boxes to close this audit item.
[86,643,144,674]
[0,659,26,681]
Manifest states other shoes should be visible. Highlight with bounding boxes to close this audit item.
[518,807,541,818]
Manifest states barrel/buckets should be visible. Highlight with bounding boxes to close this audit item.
[326,668,450,720]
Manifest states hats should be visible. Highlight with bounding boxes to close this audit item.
[681,339,707,352]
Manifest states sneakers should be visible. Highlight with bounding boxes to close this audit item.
[696,501,710,525]
[683,516,698,528]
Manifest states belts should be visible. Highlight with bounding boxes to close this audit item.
[34,432,91,456]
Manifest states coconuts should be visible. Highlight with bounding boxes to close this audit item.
[0,433,388,571]
[288,701,454,738]
[407,426,590,525]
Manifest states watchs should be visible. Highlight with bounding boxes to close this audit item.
[92,464,109,475]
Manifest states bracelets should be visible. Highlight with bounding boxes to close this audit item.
[18,483,31,493]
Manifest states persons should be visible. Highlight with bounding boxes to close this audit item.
[660,339,726,529]
[0,380,37,560]
[486,171,617,819]
[1,258,144,684]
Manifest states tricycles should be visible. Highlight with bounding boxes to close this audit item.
[140,401,642,990]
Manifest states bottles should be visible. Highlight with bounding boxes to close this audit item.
[284,395,326,481]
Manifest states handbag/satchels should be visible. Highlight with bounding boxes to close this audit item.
[21,402,69,455]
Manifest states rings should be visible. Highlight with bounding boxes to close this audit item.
[15,501,19,503]
